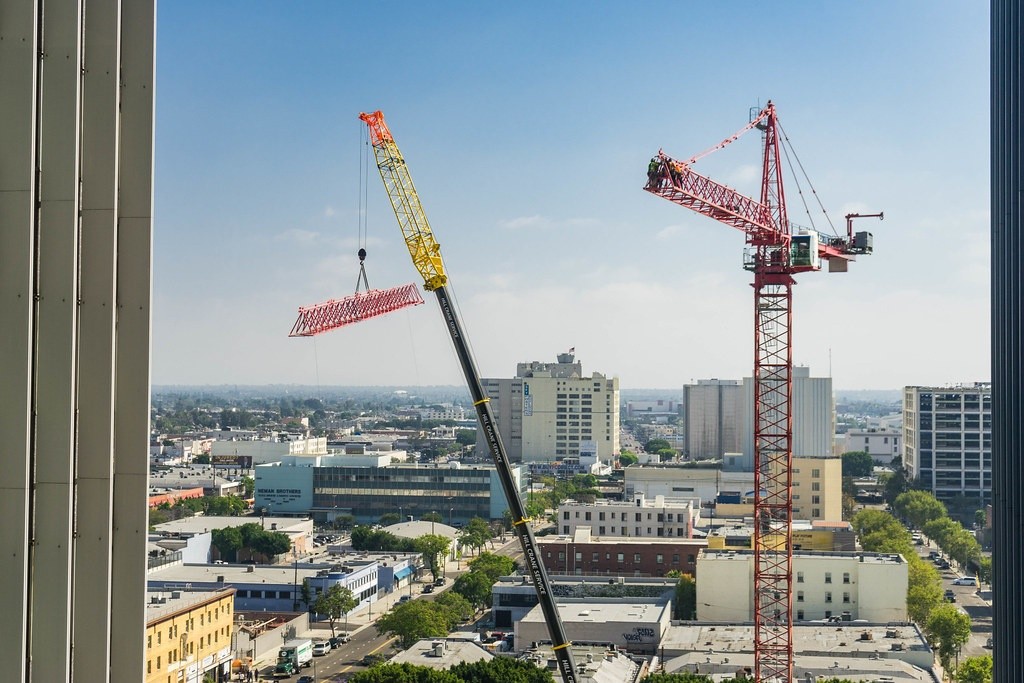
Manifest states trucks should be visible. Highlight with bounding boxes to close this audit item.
[273,638,314,678]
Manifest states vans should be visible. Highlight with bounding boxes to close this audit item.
[953,576,977,586]
[312,640,331,657]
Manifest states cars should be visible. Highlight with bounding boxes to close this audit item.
[433,578,447,586]
[398,594,411,603]
[329,633,352,649]
[943,590,957,603]
[986,637,992,648]
[929,551,950,569]
[296,675,315,683]
[313,533,340,547]
[424,584,436,593]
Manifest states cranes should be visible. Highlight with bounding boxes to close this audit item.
[641,96,887,682]
[287,109,580,683]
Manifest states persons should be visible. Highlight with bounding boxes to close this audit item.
[744,666,752,676]
[224,670,252,683]
[695,662,699,674]
[254,668,259,682]
[647,157,684,192]
